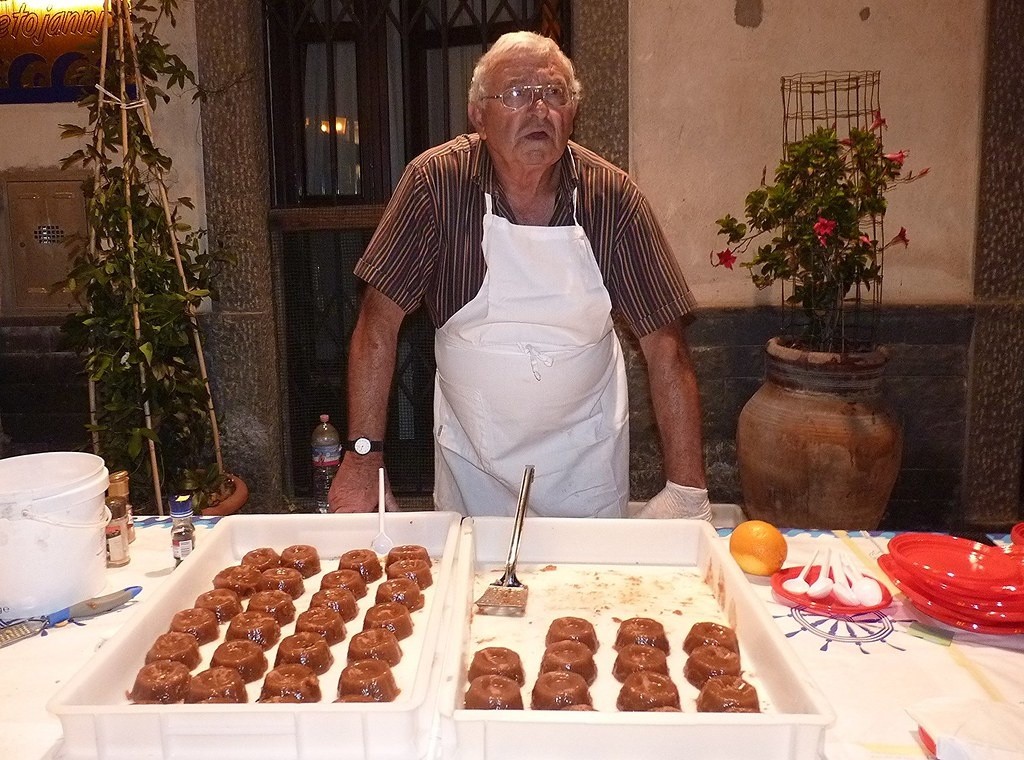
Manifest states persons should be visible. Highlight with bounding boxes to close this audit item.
[327,31,713,522]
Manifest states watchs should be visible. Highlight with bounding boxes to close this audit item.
[344,436,383,455]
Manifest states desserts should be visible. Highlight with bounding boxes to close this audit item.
[130,545,435,703]
[465,616,762,714]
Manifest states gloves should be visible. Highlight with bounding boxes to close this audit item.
[633,480,713,520]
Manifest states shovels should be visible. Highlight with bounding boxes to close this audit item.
[370,467,394,558]
[472,464,536,609]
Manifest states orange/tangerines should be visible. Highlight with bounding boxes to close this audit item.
[729,520,788,577]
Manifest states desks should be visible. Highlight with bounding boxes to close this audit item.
[0,518,1024,760]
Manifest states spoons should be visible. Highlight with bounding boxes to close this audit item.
[781,547,883,607]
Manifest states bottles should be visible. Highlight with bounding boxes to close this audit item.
[107,470,136,544]
[103,496,130,568]
[168,493,196,559]
[311,414,344,514]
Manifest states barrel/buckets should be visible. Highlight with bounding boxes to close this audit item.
[0,452,113,620]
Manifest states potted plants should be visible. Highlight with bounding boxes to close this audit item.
[53,1,249,518]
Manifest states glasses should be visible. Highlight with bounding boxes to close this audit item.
[480,84,573,110]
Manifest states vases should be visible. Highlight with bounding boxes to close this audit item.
[732,330,905,530]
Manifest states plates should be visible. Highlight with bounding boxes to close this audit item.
[877,521,1024,635]
[770,565,892,614]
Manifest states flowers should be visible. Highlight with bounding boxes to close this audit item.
[706,106,933,351]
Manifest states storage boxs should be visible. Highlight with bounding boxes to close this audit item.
[434,515,837,760]
[54,512,461,760]
